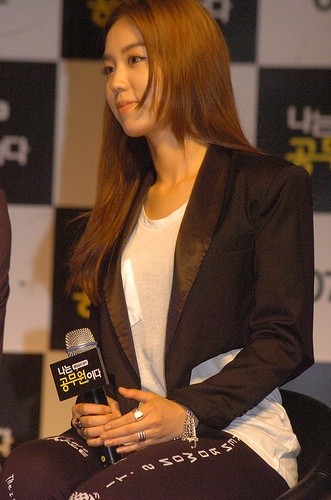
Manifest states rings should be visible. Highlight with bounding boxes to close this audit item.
[132,407,144,421]
[138,431,146,441]
[75,416,84,430]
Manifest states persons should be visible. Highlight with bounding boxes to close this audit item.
[0,0,317,500]
[0,187,13,355]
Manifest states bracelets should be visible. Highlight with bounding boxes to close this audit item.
[173,407,198,449]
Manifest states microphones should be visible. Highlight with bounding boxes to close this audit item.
[65,327,121,467]
